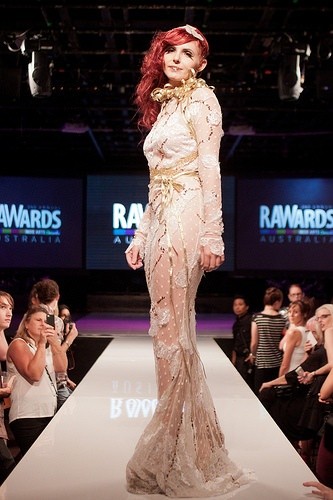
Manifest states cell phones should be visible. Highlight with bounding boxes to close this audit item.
[46,314,55,330]
[63,320,71,333]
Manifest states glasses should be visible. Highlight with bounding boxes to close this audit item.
[313,313,330,321]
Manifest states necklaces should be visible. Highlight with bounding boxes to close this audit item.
[151,68,206,102]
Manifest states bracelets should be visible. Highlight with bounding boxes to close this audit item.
[250,353,256,359]
[65,340,70,347]
[312,371,316,376]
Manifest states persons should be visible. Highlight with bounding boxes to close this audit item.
[124,24,256,498]
[230,284,333,500]
[0,278,79,486]
